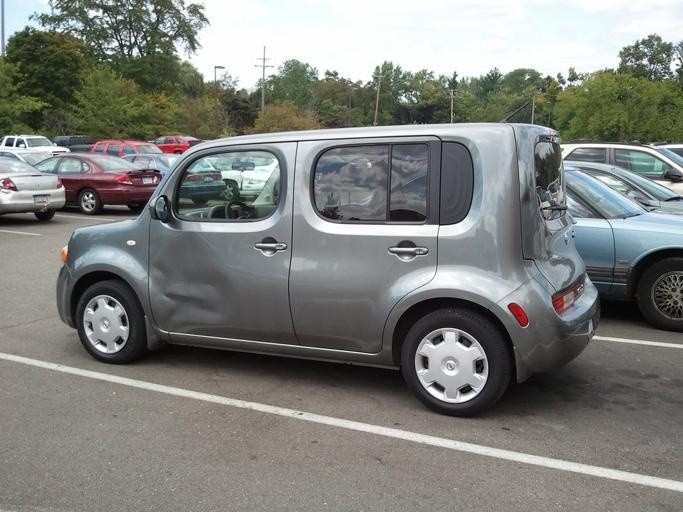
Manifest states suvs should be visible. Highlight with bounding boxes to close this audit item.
[0,134,70,158]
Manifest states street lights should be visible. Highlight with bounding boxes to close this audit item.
[215,66,226,84]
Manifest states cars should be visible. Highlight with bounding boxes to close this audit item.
[0,150,67,172]
[32,151,164,217]
[54,121,604,420]
[563,160,683,214]
[0,156,67,221]
[54,122,682,205]
[564,167,683,332]
